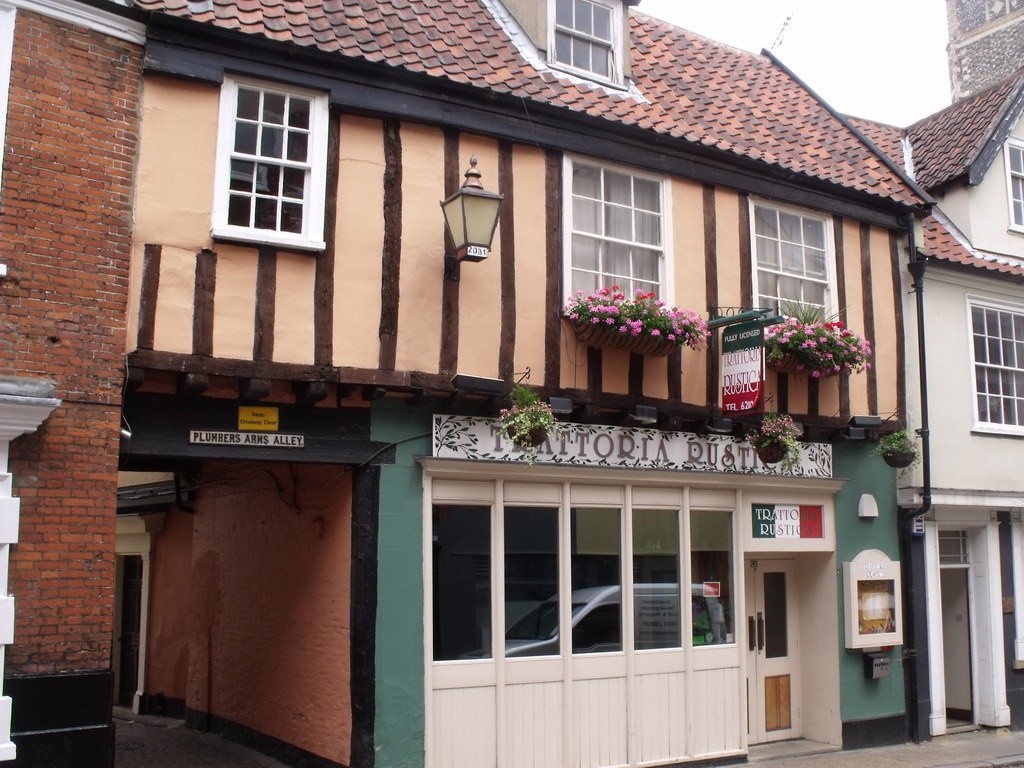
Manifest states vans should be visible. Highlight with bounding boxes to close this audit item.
[459,583,730,660]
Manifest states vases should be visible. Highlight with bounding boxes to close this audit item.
[881,451,915,468]
[766,348,830,378]
[570,318,677,356]
[507,425,548,447]
[755,440,787,463]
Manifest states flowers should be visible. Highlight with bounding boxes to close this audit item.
[745,409,803,474]
[866,430,921,481]
[761,289,875,378]
[498,381,565,468]
[565,284,711,351]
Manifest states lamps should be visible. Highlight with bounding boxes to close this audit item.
[438,154,504,263]
